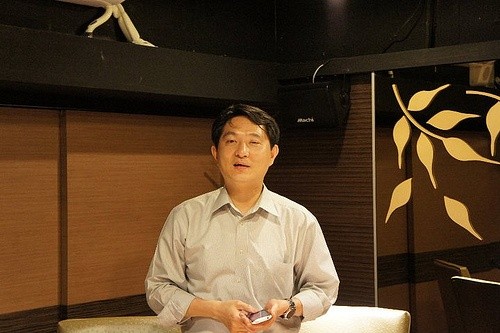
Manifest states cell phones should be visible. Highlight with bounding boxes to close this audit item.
[249,310,272,326]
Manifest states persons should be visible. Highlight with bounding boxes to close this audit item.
[143,104,340,333]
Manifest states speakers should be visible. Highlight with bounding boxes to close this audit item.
[274,76,350,132]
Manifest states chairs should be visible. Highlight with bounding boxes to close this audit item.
[430,256,471,333]
[451,275,500,333]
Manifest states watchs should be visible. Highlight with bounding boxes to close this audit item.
[280,297,297,321]
